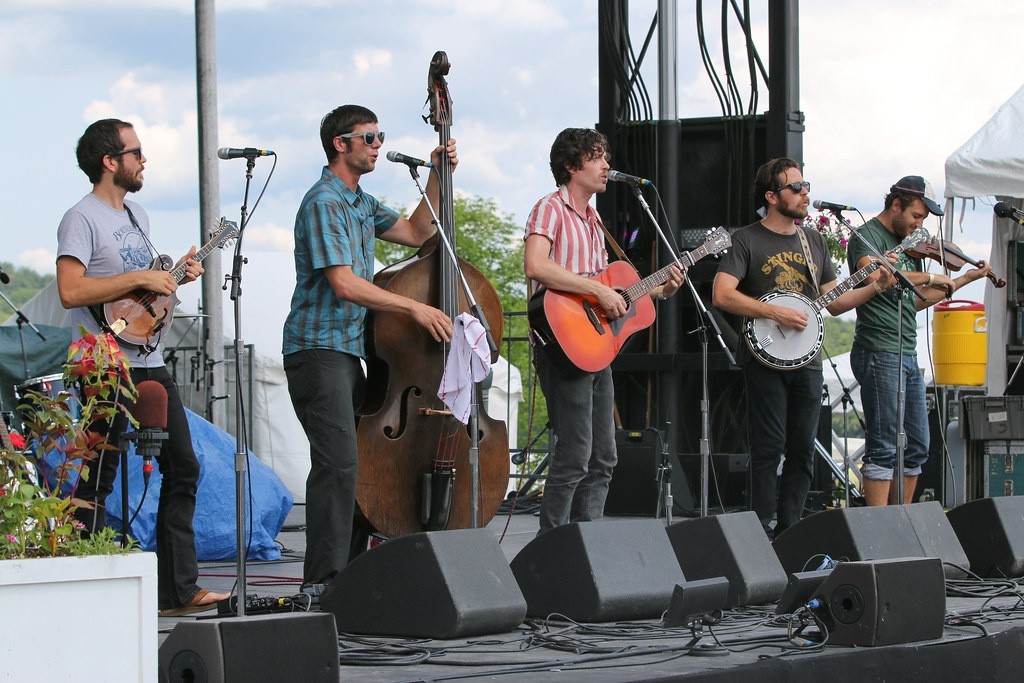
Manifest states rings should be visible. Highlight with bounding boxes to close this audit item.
[609,306,616,312]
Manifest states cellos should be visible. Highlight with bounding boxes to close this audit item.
[353,50,512,540]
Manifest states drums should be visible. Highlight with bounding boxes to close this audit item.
[16,371,86,434]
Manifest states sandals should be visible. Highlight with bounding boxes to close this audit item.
[159,589,231,617]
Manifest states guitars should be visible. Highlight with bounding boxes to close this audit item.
[526,222,733,381]
[745,226,932,370]
[102,217,239,344]
[0,412,62,550]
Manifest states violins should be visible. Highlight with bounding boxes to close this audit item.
[900,234,1006,289]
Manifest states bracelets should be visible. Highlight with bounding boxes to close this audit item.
[872,280,882,294]
[924,273,934,287]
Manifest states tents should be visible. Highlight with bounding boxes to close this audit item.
[2,211,526,528]
[935,78,1024,400]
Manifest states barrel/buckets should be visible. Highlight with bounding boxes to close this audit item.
[931,300,988,385]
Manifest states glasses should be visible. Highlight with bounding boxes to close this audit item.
[339,131,385,145]
[101,147,141,166]
[774,182,810,193]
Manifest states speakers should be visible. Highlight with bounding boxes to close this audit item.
[319,496,1024,645]
[602,430,666,517]
[158,612,340,683]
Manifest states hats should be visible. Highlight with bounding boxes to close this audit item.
[892,175,944,216]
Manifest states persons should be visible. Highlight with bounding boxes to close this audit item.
[712,157,899,537]
[280,107,458,612]
[521,126,684,536]
[848,176,993,510]
[55,118,236,617]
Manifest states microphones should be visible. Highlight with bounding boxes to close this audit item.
[813,200,856,210]
[386,151,436,169]
[131,381,168,486]
[993,201,1024,227]
[607,170,650,185]
[218,147,275,160]
